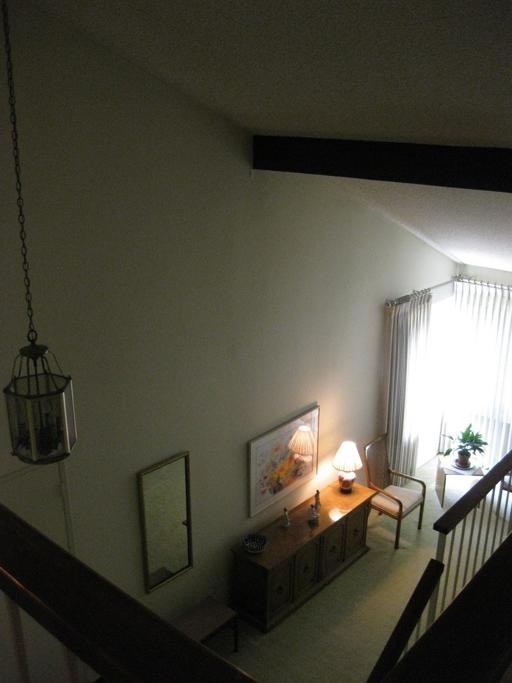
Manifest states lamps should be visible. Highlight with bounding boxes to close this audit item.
[332,440,364,493]
[289,424,317,463]
[1,1,75,464]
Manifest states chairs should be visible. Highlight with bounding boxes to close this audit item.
[364,432,426,550]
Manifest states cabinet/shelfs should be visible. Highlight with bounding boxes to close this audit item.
[227,479,379,633]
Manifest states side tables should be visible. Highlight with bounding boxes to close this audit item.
[176,596,239,653]
[435,457,485,508]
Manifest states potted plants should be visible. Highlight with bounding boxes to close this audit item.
[455,424,489,468]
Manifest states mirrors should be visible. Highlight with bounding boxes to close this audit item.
[137,451,193,594]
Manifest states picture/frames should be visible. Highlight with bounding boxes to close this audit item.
[246,402,320,519]
